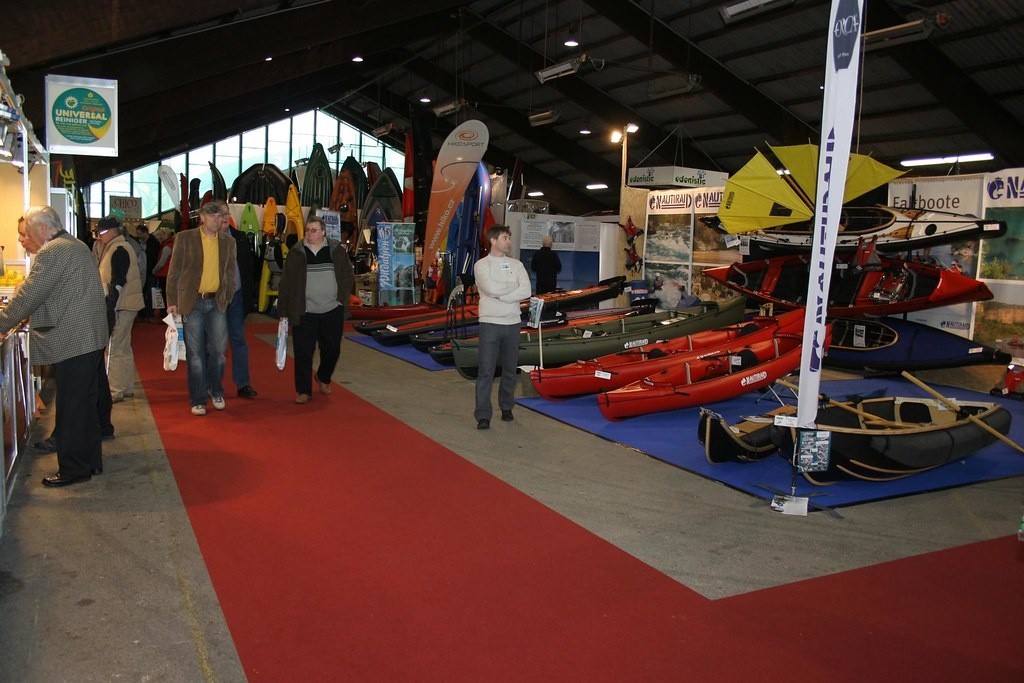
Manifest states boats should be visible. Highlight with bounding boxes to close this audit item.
[168,100,1011,487]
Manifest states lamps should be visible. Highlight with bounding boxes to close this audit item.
[718,0,797,24]
[327,142,344,154]
[372,124,394,138]
[528,110,561,127]
[433,102,462,118]
[534,58,579,85]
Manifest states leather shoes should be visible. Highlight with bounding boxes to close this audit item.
[90,468,103,474]
[42,472,91,487]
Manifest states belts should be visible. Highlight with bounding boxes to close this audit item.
[198,293,215,300]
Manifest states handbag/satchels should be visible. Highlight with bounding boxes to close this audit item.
[151,287,165,308]
[276,318,288,370]
[162,313,186,371]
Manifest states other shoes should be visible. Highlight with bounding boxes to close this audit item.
[31,439,58,451]
[102,432,114,439]
[315,375,331,394]
[191,404,206,415]
[478,419,490,429]
[208,389,225,409]
[237,386,257,397]
[501,410,513,421]
[112,396,123,403]
[124,392,134,397]
[296,394,311,404]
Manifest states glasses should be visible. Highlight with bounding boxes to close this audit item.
[304,228,322,233]
[98,230,110,237]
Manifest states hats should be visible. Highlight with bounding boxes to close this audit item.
[92,217,120,231]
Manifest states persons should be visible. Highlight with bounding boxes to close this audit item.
[276,216,353,404]
[474,224,532,430]
[0,204,114,488]
[91,214,175,405]
[424,258,441,306]
[165,201,258,416]
[530,234,562,295]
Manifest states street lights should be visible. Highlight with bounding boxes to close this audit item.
[609,122,640,308]
